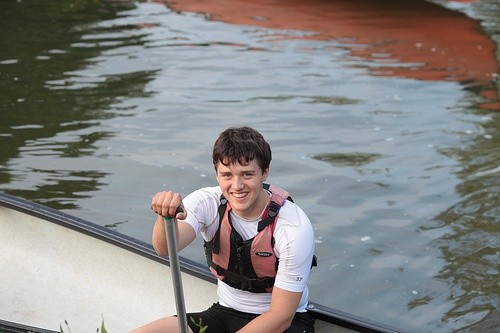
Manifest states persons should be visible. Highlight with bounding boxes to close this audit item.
[131,126,317,333]
